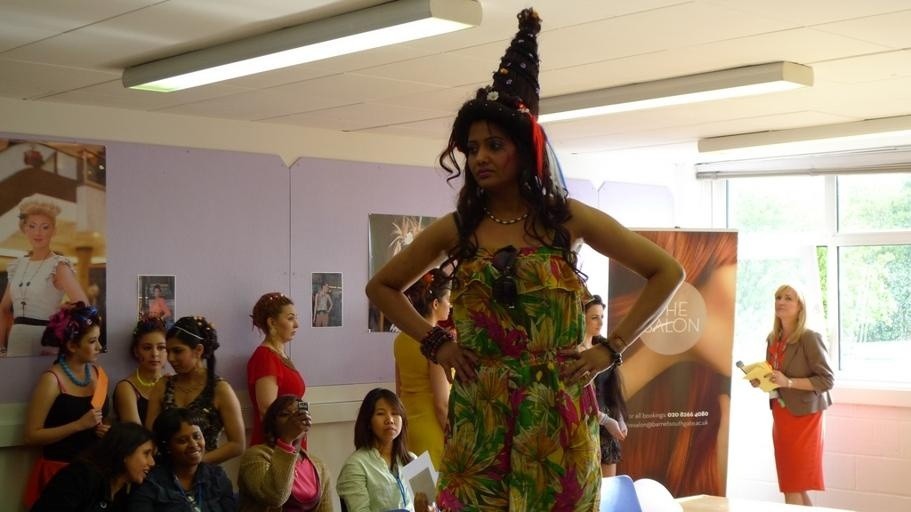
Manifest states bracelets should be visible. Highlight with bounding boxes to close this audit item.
[598,413,609,426]
[592,334,622,373]
[419,325,453,365]
[787,378,792,389]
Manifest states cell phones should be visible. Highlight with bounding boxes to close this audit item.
[298,401,311,421]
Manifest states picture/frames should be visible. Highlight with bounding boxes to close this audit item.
[310,272,343,328]
[137,274,175,329]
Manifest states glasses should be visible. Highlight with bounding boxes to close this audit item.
[491,245,516,310]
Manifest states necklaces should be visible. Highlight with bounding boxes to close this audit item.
[136,367,162,387]
[60,354,91,387]
[19,252,52,306]
[481,203,532,225]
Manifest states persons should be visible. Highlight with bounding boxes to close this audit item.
[393,268,453,472]
[139,408,236,512]
[69,422,156,512]
[312,281,333,326]
[246,292,308,452]
[364,87,686,512]
[112,316,168,433]
[578,294,629,478]
[335,388,429,512]
[24,300,112,509]
[236,395,333,512]
[148,283,171,322]
[0,200,91,356]
[750,283,836,507]
[145,316,246,465]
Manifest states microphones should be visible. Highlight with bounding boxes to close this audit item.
[735,359,747,375]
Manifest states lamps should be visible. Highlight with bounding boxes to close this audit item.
[538,60,814,124]
[122,0,481,94]
[698,114,911,155]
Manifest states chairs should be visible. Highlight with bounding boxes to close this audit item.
[599,475,642,511]
[634,478,684,511]
